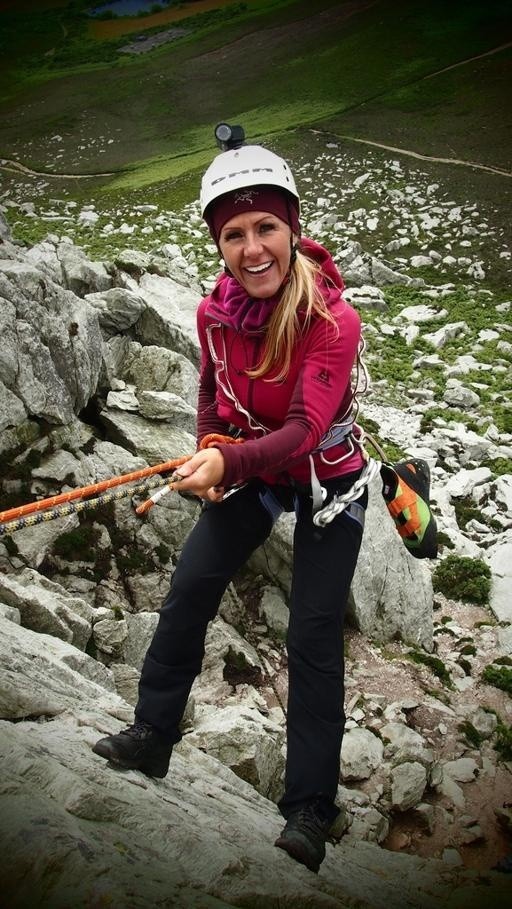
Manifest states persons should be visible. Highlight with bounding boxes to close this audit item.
[92,144,370,874]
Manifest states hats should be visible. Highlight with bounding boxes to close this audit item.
[208,185,300,239]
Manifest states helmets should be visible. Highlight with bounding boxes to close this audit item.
[199,145,302,221]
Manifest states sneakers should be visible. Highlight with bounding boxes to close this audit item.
[381,458,438,559]
[93,722,174,778]
[275,792,341,873]
